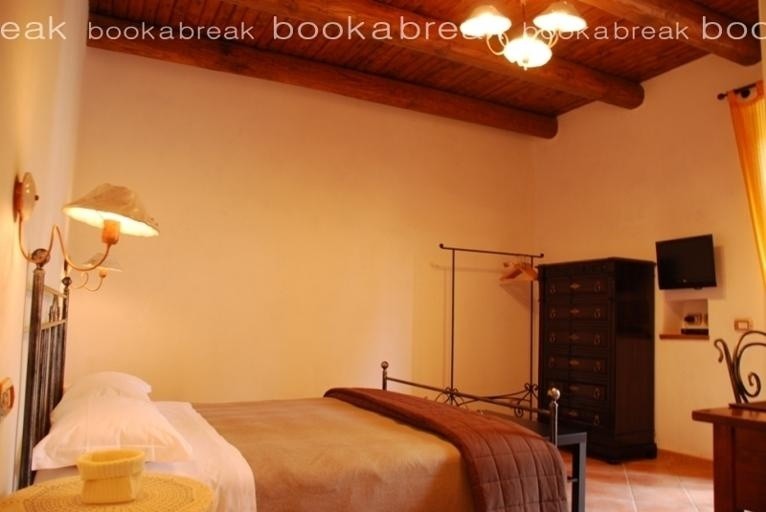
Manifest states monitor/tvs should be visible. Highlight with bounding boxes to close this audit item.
[656,232,719,291]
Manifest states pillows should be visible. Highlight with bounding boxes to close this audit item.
[50,371,152,424]
[31,397,193,472]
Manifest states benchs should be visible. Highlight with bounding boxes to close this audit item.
[481,411,588,511]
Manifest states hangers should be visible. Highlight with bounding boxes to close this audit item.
[501,254,538,282]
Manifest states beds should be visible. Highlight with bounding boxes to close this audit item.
[19,249,561,512]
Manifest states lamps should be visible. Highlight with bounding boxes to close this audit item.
[15,172,160,272]
[65,255,124,292]
[459,0,587,68]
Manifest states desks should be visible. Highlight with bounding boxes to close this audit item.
[692,401,766,512]
[0,476,213,512]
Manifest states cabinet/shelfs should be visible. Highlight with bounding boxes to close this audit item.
[535,257,657,464]
